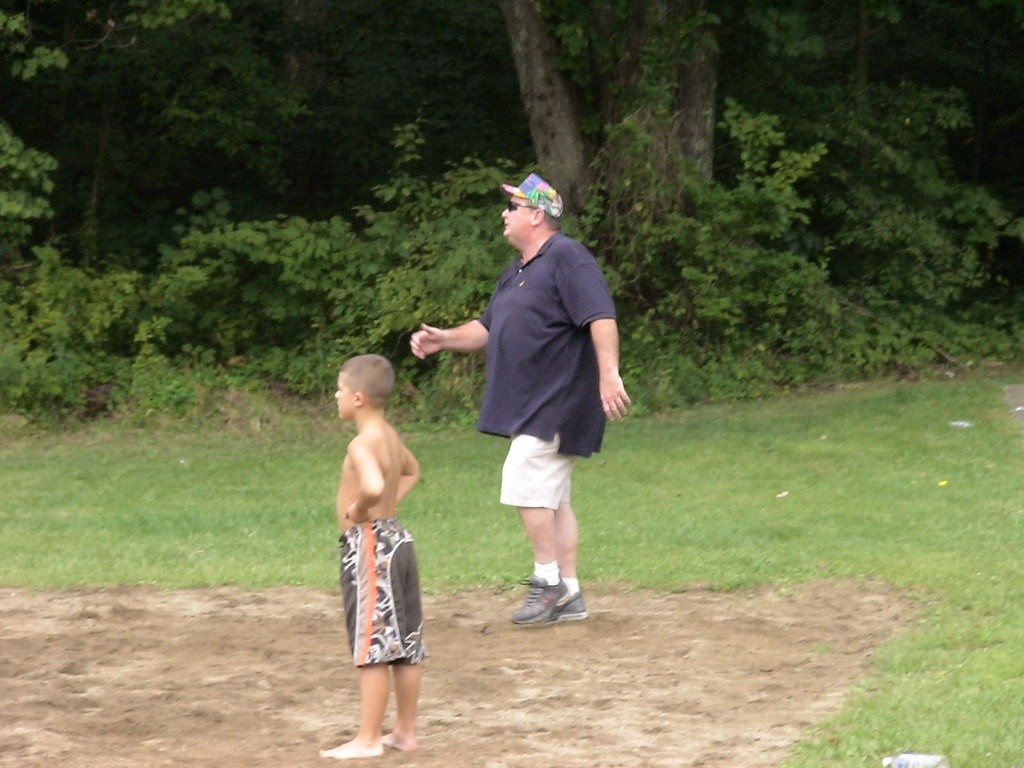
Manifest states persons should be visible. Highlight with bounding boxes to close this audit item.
[320,353,425,759]
[410,173,632,628]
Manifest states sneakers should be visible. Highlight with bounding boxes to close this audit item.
[542,589,589,625]
[510,575,568,625]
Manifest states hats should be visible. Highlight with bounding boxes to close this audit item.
[501,172,563,218]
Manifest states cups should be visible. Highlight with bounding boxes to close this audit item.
[882,753,949,768]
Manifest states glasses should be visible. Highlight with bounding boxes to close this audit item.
[507,200,539,212]
